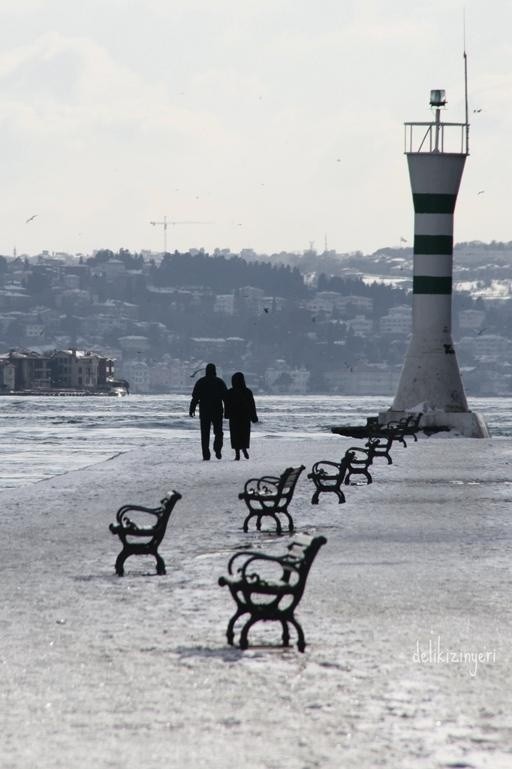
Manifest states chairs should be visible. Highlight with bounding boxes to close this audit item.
[210,414,423,652]
[108,489,181,576]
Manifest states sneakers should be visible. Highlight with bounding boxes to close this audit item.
[203,449,249,460]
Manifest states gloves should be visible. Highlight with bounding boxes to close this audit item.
[190,410,195,417]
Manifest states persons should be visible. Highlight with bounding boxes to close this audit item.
[189,364,228,460]
[224,372,257,460]
